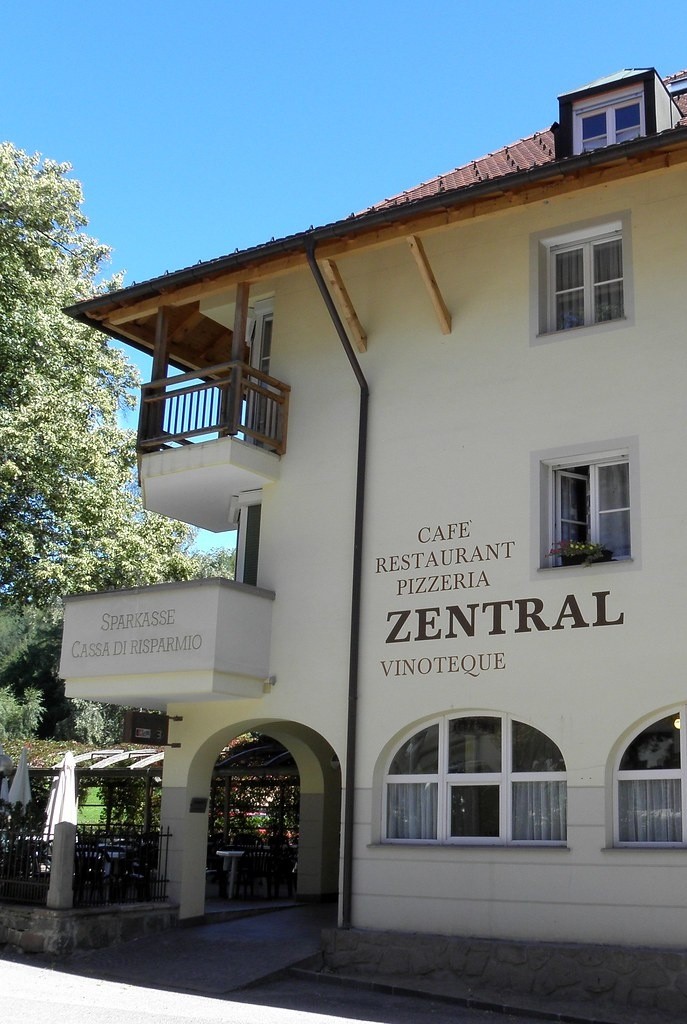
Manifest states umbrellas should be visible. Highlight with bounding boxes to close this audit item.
[36,750,79,844]
[8,745,32,815]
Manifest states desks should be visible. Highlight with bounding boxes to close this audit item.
[74,850,127,884]
[216,849,273,899]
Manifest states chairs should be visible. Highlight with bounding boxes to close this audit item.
[5,828,299,902]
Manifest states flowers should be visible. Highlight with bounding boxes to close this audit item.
[544,539,606,568]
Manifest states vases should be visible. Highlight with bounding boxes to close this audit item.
[559,553,591,566]
